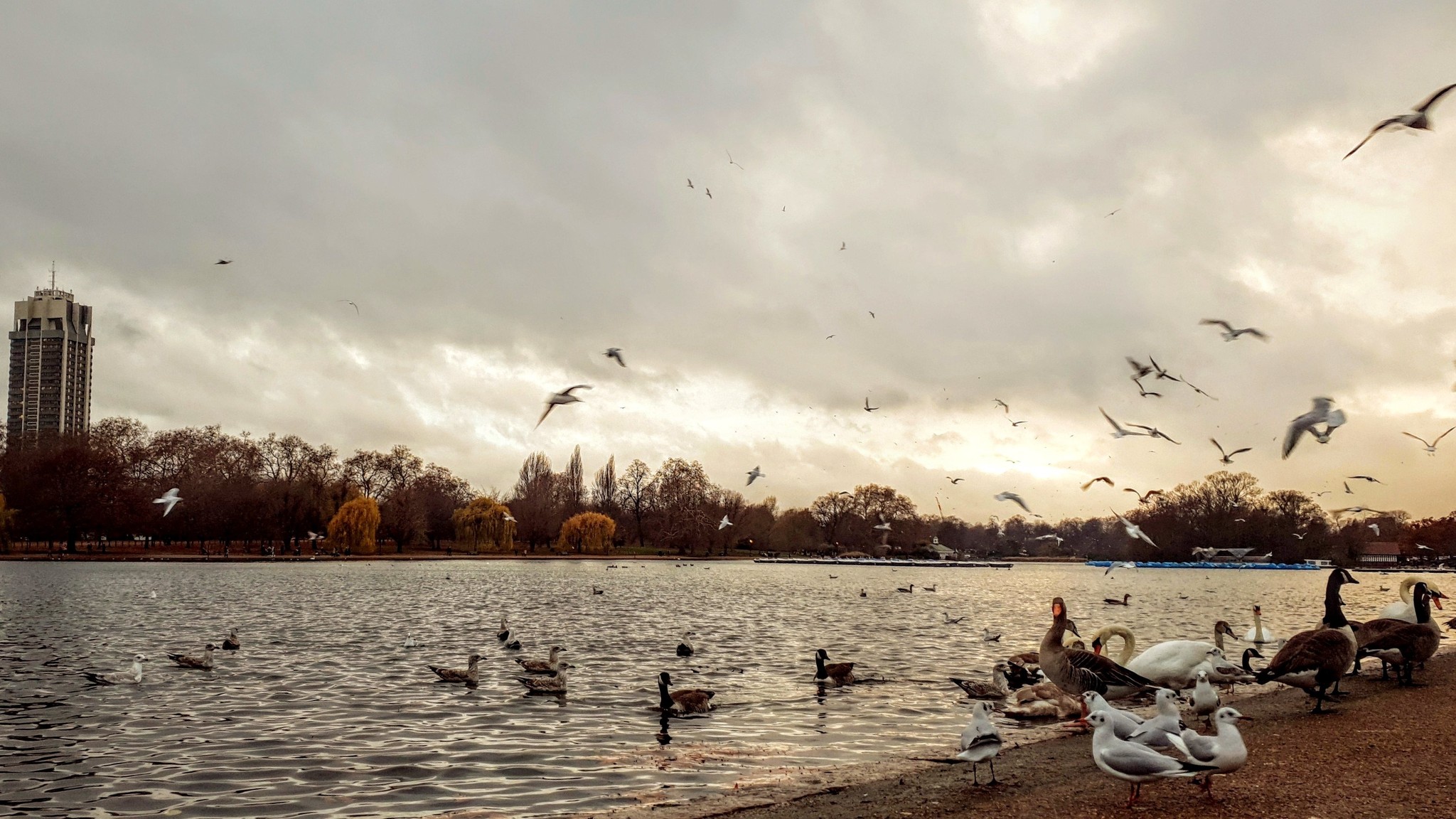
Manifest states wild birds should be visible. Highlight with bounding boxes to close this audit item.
[123,84,1456,809]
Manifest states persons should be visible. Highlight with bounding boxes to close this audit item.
[657,549,671,558]
[446,546,452,556]
[761,548,838,560]
[47,543,106,558]
[514,547,527,557]
[202,544,352,557]
